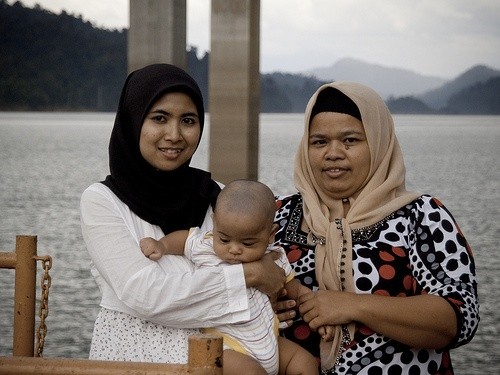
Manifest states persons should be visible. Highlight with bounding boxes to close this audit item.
[271,80,480,375]
[77,63,285,366]
[140,180,336,375]
